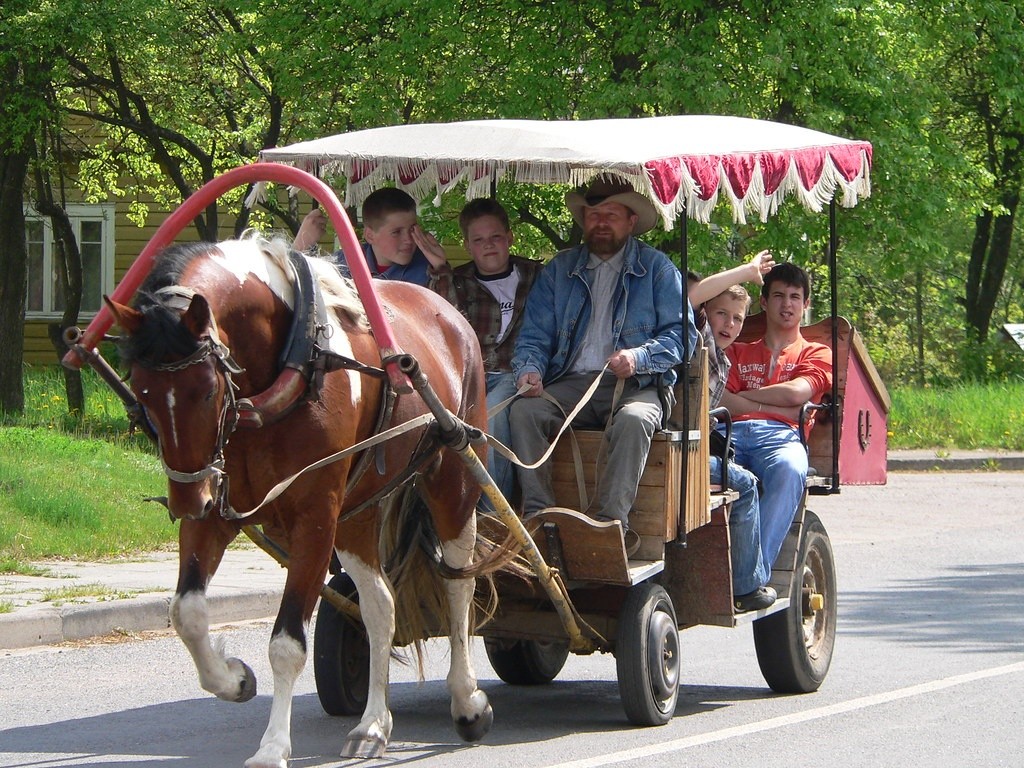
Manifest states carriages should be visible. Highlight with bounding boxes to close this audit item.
[60,114,891,768]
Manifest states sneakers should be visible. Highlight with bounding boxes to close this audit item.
[734,586,777,613]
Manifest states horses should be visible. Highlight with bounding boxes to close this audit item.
[102,229,493,768]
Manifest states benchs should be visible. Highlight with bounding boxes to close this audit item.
[721,314,854,495]
[506,348,712,564]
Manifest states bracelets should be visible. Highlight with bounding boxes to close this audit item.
[758,403,762,414]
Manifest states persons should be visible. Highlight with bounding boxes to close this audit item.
[289,173,835,614]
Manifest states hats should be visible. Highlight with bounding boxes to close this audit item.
[565,171,658,235]
